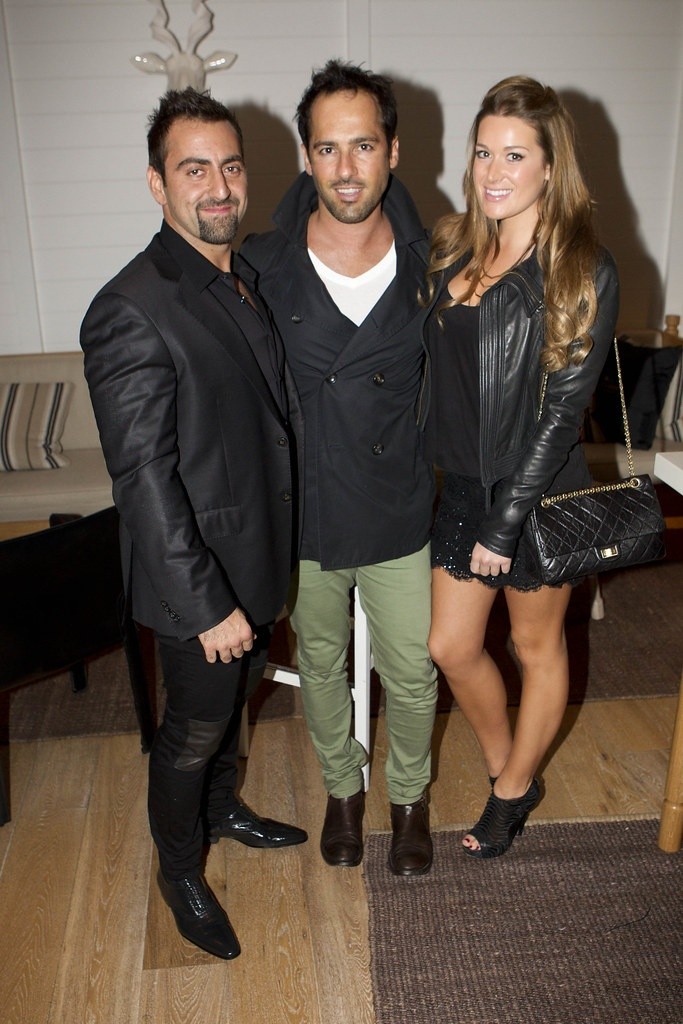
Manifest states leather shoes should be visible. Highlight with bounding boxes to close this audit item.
[156,868,241,960]
[388,796,434,875]
[205,805,308,849]
[320,790,367,866]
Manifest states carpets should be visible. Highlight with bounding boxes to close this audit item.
[7,618,303,744]
[362,811,683,1023]
[365,477,683,720]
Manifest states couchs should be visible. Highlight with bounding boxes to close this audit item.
[1,353,115,521]
[580,315,683,483]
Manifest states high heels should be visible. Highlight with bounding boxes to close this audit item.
[462,777,541,858]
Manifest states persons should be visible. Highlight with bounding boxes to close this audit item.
[80,89,311,958]
[421,77,619,858]
[239,63,441,872]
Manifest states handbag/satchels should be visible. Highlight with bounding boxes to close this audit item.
[524,475,668,586]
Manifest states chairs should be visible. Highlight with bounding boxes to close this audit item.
[0,505,157,827]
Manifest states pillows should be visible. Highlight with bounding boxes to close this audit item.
[0,381,74,473]
[588,339,683,450]
